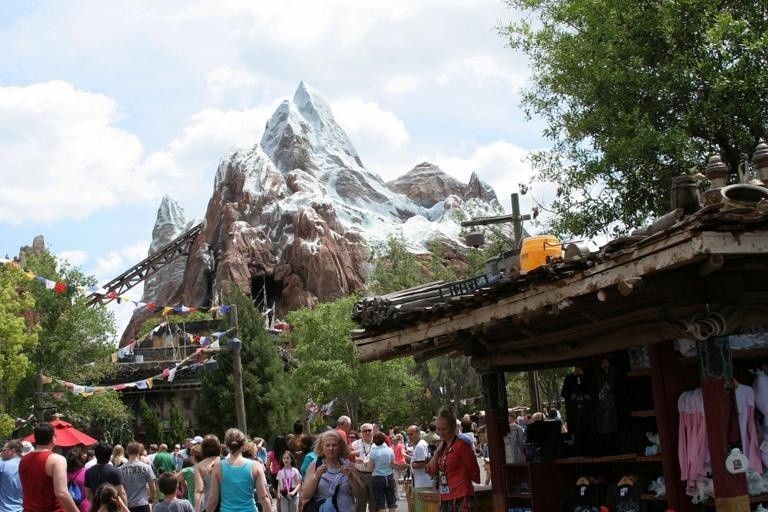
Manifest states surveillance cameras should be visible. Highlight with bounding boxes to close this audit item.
[466,232,485,248]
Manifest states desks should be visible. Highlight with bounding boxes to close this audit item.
[414,486,494,512]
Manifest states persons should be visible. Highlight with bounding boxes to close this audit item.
[386,416,441,511]
[180,428,273,511]
[266,415,367,511]
[351,423,398,511]
[78,442,193,511]
[469,404,566,463]
[425,404,481,511]
[2,421,80,512]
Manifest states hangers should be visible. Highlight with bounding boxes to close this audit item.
[734,377,739,388]
[574,470,640,486]
[573,366,585,375]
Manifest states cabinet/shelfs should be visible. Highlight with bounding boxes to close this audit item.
[626,368,668,504]
[501,461,578,512]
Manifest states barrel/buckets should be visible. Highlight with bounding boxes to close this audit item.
[520,234,562,272]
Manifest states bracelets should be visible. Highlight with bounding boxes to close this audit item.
[314,473,320,481]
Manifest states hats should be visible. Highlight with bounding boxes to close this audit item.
[190,436,204,444]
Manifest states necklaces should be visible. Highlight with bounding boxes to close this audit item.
[326,458,343,474]
